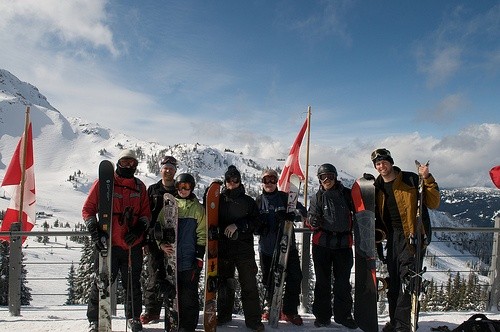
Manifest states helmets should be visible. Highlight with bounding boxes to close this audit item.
[175,173,195,191]
[318,163,338,178]
[117,149,139,171]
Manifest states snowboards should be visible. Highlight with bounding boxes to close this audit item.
[351,176,379,332]
[203,182,219,332]
[164,193,179,332]
[98,159,115,332]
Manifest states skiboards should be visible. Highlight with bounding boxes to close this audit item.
[268,173,301,328]
[411,160,430,332]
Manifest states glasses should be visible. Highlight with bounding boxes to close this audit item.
[262,175,278,184]
[227,177,239,183]
[371,150,388,160]
[319,174,334,181]
[162,156,177,165]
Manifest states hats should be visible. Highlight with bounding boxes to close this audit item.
[224,165,241,179]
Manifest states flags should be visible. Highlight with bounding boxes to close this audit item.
[0,122,36,249]
[278,119,309,194]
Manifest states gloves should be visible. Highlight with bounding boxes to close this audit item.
[85,217,108,252]
[378,245,385,261]
[275,211,295,223]
[124,216,150,245]
[364,173,375,182]
[191,258,202,272]
[310,215,321,227]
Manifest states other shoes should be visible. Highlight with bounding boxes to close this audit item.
[128,320,143,332]
[382,322,407,332]
[334,315,358,330]
[285,315,303,326]
[90,322,98,332]
[141,314,160,323]
[246,323,264,330]
[215,315,232,325]
[314,316,331,327]
[261,311,285,320]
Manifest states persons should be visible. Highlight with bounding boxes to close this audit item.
[82,149,151,332]
[306,163,359,328]
[252,170,307,325]
[154,173,208,332]
[371,148,440,330]
[139,157,197,324]
[203,164,266,331]
